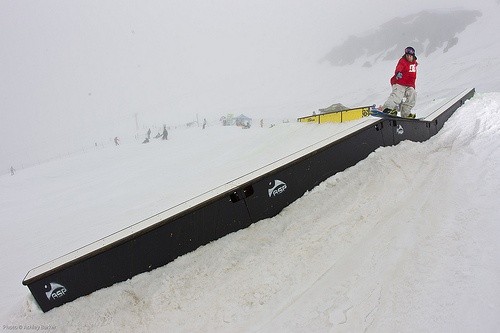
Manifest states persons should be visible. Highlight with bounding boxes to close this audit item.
[11,165,16,176]
[114,136,120,146]
[154,127,168,140]
[203,118,206,129]
[147,128,151,138]
[382,46,418,117]
[261,118,264,127]
[246,122,251,128]
[142,137,149,143]
[372,104,382,111]
[269,124,272,128]
[398,99,403,111]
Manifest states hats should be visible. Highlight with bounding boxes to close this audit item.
[402,47,417,60]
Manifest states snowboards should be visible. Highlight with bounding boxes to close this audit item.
[371,111,425,120]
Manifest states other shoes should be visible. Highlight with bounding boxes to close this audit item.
[407,114,413,117]
[383,109,393,113]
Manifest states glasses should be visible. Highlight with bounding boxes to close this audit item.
[405,49,414,54]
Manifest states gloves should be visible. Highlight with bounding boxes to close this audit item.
[396,72,402,80]
